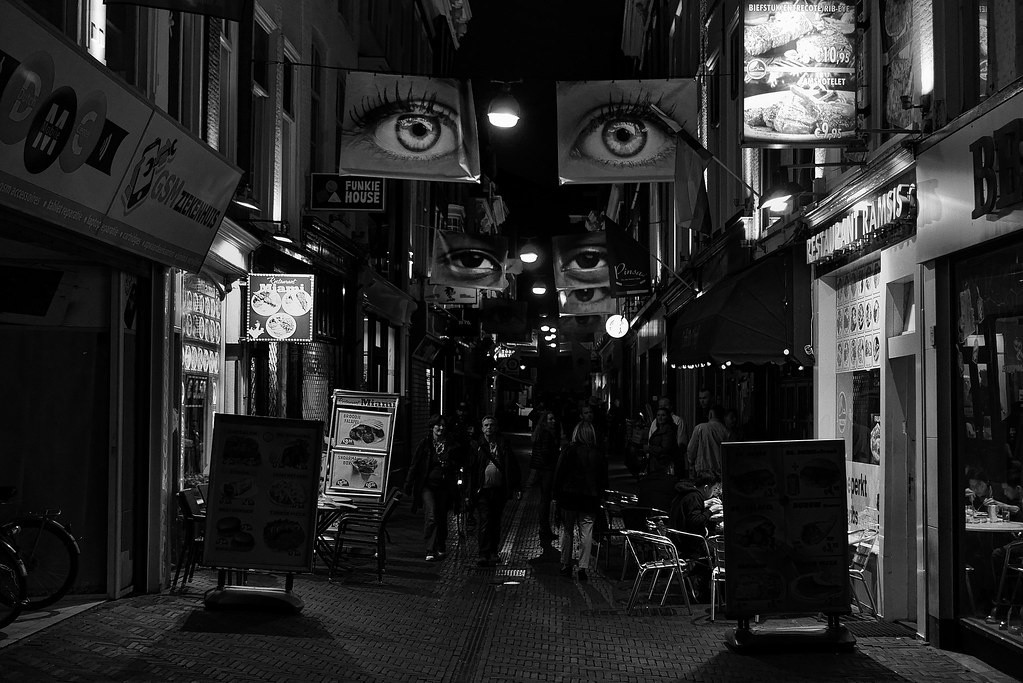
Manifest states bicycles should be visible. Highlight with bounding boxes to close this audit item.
[449,460,479,538]
[0,485,83,630]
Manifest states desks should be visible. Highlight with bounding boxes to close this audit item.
[317,492,354,559]
[965,508,1023,618]
[314,501,353,576]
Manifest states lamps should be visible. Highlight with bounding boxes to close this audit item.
[519,237,539,265]
[245,214,295,245]
[531,274,548,295]
[482,76,527,133]
[765,185,829,217]
[901,95,935,115]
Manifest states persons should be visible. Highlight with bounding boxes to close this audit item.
[405,415,524,566]
[530,398,626,582]
[964,466,1023,615]
[645,389,735,555]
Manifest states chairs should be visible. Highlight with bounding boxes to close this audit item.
[170,478,402,593]
[600,487,727,621]
[817,527,878,622]
[985,540,1023,635]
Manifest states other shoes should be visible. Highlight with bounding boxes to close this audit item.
[437,551,445,556]
[487,553,502,563]
[560,563,570,575]
[425,555,435,561]
